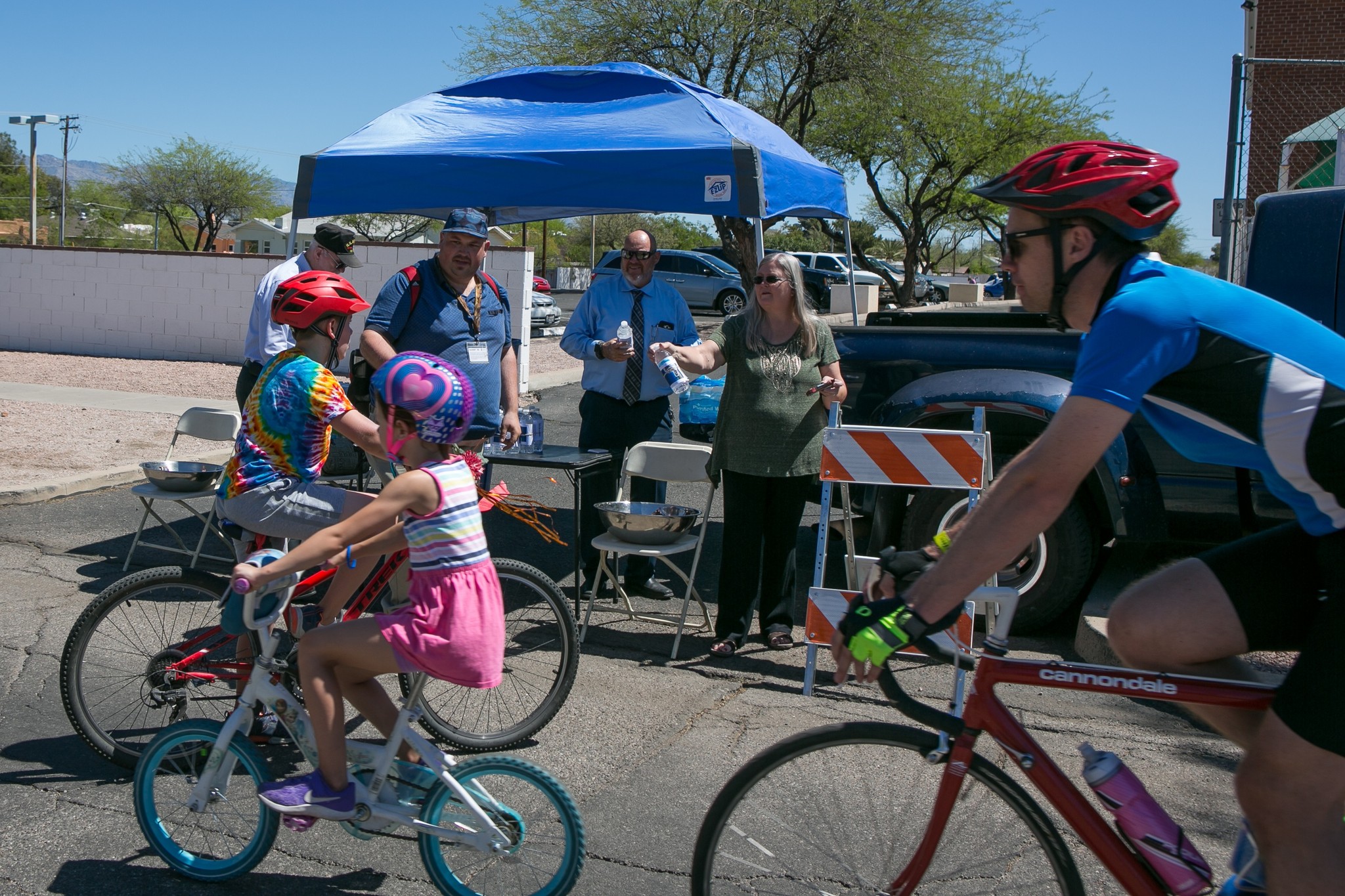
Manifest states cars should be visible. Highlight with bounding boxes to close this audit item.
[530,291,561,328]
[983,273,1010,298]
[533,275,552,295]
[834,251,952,304]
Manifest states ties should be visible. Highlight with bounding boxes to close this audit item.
[623,290,644,406]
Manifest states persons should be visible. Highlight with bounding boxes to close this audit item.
[214,205,850,820]
[808,143,1344,896]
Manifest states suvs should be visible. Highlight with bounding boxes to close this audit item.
[590,249,748,316]
[784,249,899,300]
[690,246,848,314]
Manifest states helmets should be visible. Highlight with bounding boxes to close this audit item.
[369,350,476,444]
[270,270,371,328]
[969,141,1180,241]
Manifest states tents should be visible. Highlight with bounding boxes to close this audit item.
[286,60,858,335]
[1275,105,1344,190]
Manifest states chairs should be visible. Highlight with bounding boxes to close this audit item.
[123,407,236,572]
[580,441,715,659]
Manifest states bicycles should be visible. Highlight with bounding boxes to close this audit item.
[131,550,587,896]
[687,588,1286,896]
[58,515,581,777]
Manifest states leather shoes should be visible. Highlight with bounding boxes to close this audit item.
[623,575,674,599]
[580,579,605,597]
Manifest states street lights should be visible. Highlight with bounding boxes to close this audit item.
[9,114,59,245]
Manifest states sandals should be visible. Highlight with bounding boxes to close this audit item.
[768,633,794,648]
[710,638,735,655]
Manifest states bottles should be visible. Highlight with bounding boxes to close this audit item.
[653,343,689,394]
[617,321,633,355]
[482,406,544,455]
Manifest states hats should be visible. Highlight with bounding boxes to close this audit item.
[441,207,489,239]
[315,223,364,268]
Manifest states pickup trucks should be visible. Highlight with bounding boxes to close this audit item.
[808,187,1345,643]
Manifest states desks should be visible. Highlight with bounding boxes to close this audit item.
[482,445,618,620]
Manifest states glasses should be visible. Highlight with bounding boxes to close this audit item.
[317,246,348,269]
[621,248,654,260]
[753,275,784,284]
[648,324,659,347]
[1006,224,1076,258]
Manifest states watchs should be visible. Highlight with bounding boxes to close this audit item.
[594,341,606,360]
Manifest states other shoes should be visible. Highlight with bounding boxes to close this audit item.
[223,709,296,744]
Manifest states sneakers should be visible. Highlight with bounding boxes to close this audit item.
[258,768,356,821]
[397,745,442,796]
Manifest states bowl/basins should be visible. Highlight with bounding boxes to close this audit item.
[594,501,702,545]
[138,461,226,492]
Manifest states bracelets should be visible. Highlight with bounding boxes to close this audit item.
[346,545,356,569]
[933,533,954,557]
